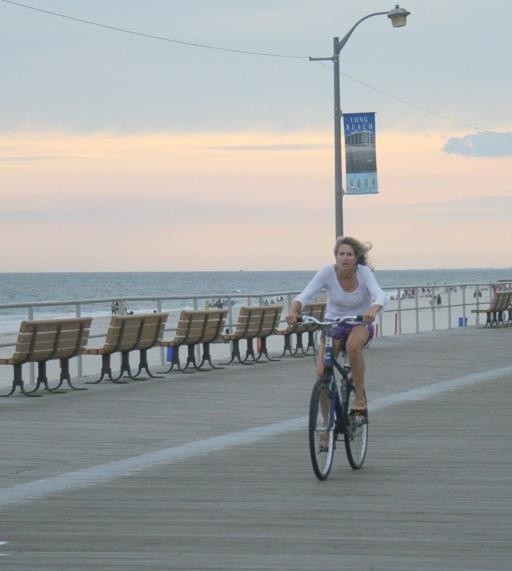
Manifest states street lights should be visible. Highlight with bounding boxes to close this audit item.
[333,3,411,238]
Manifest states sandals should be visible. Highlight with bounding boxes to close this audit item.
[350,400,367,414]
[319,427,337,452]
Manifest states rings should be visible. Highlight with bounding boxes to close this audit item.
[289,319,290,321]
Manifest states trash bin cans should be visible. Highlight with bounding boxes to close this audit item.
[458,317,468,327]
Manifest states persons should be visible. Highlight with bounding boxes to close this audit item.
[286,235,387,450]
[114,301,119,316]
[111,301,115,316]
[436,294,441,304]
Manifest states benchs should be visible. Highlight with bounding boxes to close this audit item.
[271,303,326,358]
[79,312,167,384]
[471,290,512,328]
[213,305,283,365]
[0,317,92,397]
[157,310,229,374]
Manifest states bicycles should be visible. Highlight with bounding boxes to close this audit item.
[285,315,370,480]
